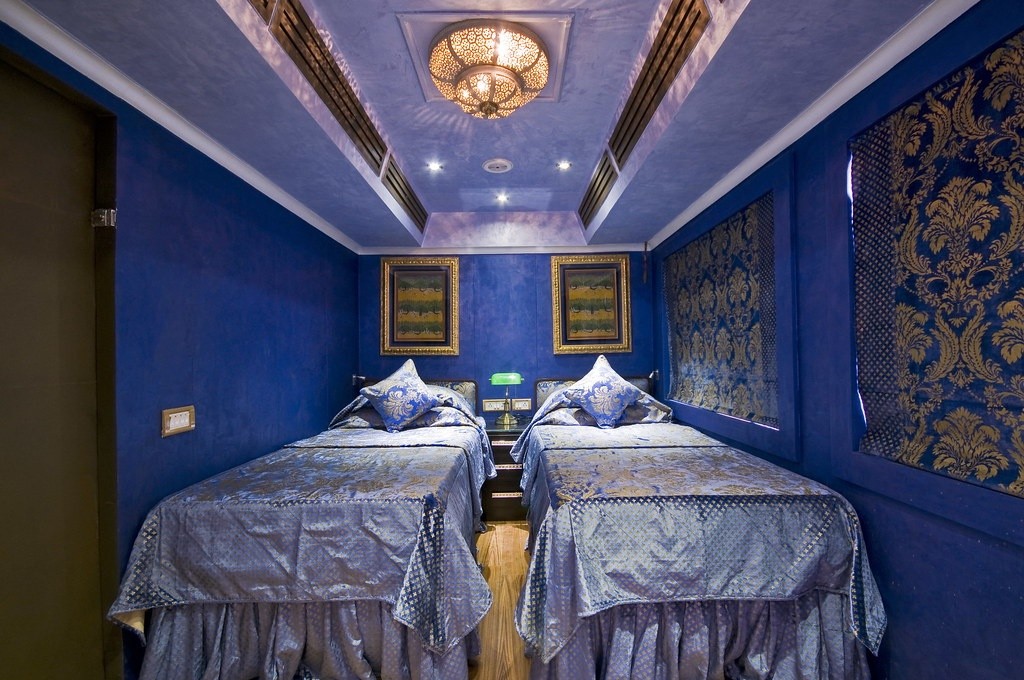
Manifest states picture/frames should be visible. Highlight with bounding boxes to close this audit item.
[549,252,633,356]
[380,256,460,357]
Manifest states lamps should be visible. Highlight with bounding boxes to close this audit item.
[429,20,551,119]
[492,372,521,426]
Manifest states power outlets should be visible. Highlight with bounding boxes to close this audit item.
[161,404,196,437]
[481,399,531,411]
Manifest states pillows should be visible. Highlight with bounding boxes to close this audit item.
[535,406,670,425]
[360,386,453,408]
[347,411,467,431]
[363,358,442,429]
[550,384,648,408]
[566,349,648,431]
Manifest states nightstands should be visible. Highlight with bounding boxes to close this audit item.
[481,420,530,521]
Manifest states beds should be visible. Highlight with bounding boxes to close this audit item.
[528,378,889,680]
[100,379,496,680]
